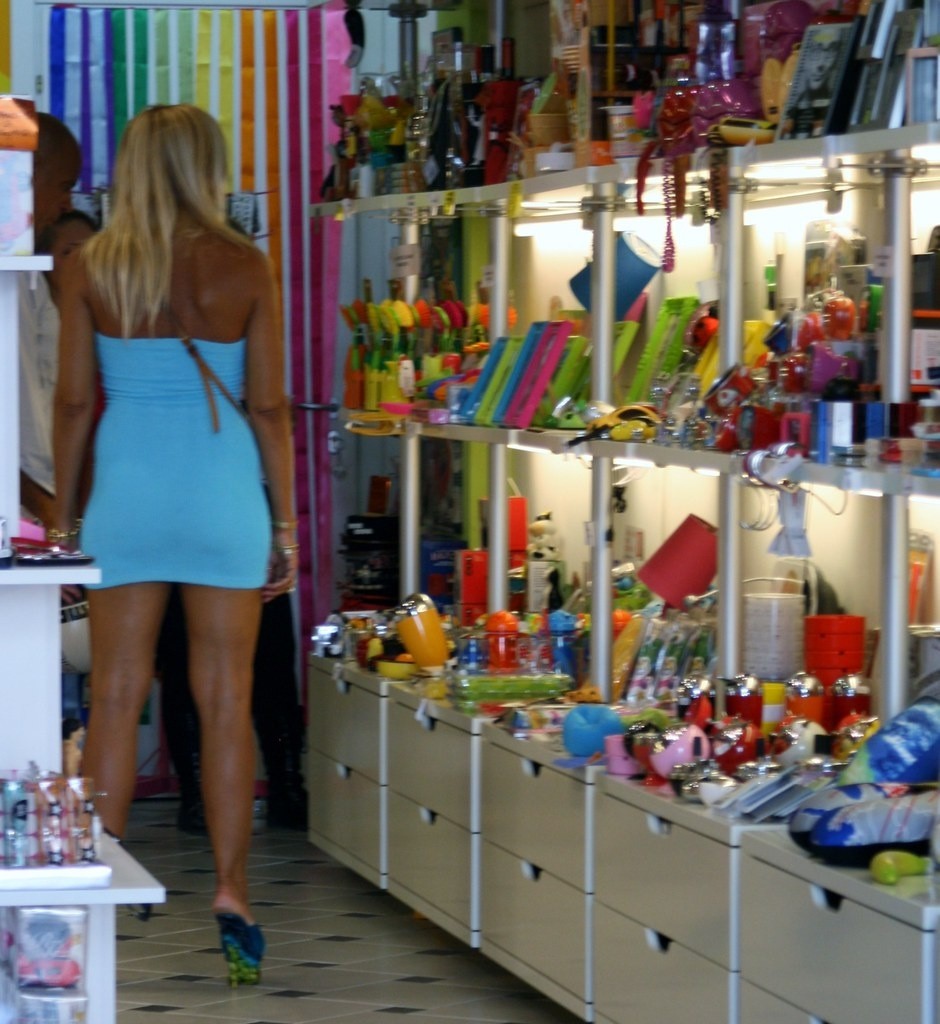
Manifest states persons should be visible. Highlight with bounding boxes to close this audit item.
[34,114,81,249]
[781,27,840,140]
[30,210,98,405]
[44,104,301,985]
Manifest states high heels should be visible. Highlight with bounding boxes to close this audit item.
[216,914,267,988]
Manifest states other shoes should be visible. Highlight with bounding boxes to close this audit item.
[179,801,207,834]
[267,782,308,832]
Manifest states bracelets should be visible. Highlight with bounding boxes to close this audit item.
[49,526,80,543]
[272,544,298,555]
[272,518,299,530]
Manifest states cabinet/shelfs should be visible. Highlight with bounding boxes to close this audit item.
[309,124,940,1024]
[0,150,167,1024]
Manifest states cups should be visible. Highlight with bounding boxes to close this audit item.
[604,735,642,774]
[395,608,449,669]
[455,633,540,674]
[525,113,569,147]
[597,105,651,163]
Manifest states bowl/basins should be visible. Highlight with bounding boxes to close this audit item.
[373,660,419,681]
